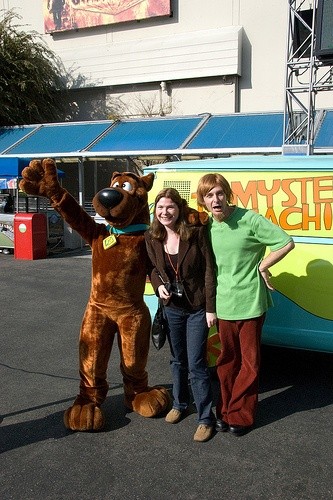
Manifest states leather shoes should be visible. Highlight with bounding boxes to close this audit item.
[229,425,249,435]
[214,418,229,432]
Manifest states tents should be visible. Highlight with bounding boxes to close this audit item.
[0,158,63,213]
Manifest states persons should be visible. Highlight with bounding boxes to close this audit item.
[198,173,294,435]
[145,189,217,441]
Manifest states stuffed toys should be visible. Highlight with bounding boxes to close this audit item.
[20,159,208,430]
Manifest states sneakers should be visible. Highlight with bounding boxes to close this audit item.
[165,408,182,423]
[194,424,211,442]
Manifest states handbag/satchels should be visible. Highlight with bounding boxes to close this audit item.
[152,292,166,349]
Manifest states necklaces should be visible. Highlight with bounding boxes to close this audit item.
[102,227,120,250]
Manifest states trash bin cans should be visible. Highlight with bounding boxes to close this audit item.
[13,212,48,261]
